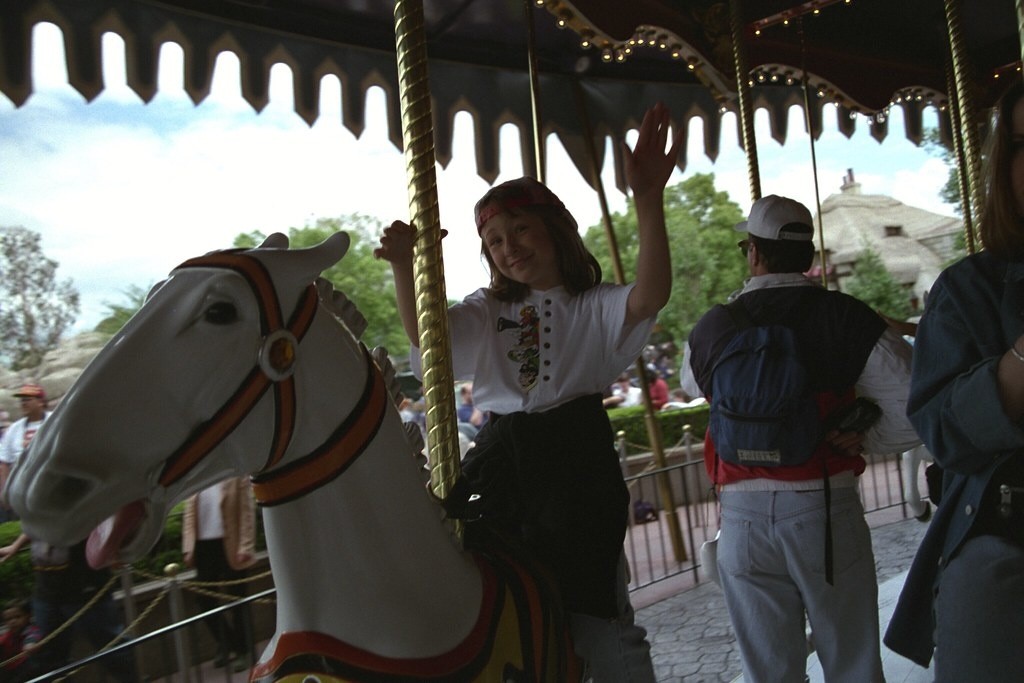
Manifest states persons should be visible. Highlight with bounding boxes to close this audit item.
[883,65,1024,683]
[399,367,691,442]
[0,531,139,683]
[0,382,56,507]
[374,103,685,683]
[877,309,919,337]
[0,596,43,683]
[679,194,925,683]
[181,475,259,672]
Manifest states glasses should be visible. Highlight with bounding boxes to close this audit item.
[738,239,753,256]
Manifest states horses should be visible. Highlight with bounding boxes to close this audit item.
[1,230,587,683]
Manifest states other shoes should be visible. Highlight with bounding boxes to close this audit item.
[231,652,254,671]
[217,650,238,667]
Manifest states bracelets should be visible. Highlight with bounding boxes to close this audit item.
[1011,348,1024,362]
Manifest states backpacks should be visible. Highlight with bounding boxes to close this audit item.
[708,286,840,467]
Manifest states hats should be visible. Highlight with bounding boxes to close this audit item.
[474,176,562,238]
[734,195,814,241]
[13,383,45,399]
